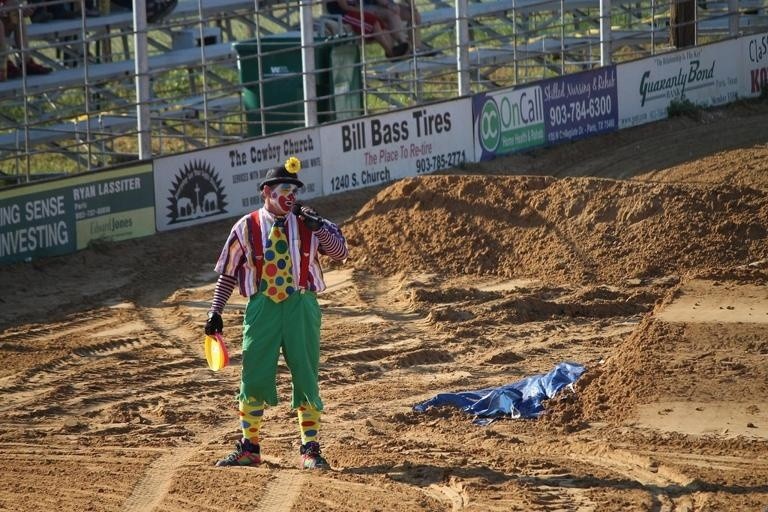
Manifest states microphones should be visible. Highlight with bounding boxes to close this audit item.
[291,204,322,223]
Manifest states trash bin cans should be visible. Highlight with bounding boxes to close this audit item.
[230,30,365,137]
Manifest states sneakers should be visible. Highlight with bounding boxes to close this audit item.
[299,443,329,468]
[216,440,262,466]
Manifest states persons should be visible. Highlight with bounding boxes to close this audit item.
[202,156,350,473]
[81,1,180,25]
[0,0,55,83]
[365,1,441,58]
[28,1,100,56]
[326,0,412,62]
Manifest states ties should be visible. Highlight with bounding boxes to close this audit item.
[261,216,296,302]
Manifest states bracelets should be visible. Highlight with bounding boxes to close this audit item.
[206,310,221,319]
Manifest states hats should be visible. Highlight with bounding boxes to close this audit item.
[260,166,304,189]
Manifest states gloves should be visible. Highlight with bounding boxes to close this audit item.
[203,313,225,334]
[299,208,328,229]
